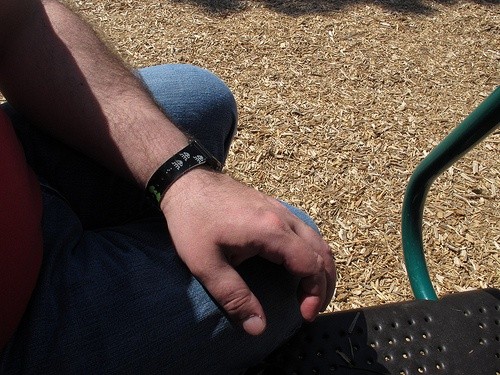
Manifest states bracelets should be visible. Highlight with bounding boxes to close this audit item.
[143,134,222,210]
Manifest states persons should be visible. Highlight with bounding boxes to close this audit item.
[0,0,341,375]
